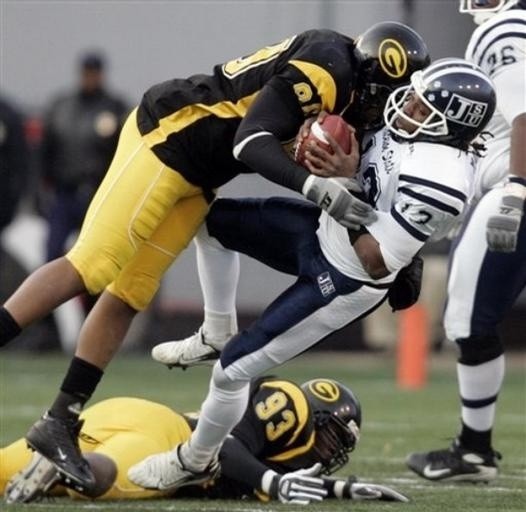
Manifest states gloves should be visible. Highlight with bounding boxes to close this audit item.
[344,481,406,501]
[270,462,329,505]
[484,195,523,252]
[306,175,372,231]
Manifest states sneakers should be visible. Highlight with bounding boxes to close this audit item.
[27,413,99,488]
[405,440,499,483]
[127,444,220,491]
[4,453,56,505]
[150,327,236,369]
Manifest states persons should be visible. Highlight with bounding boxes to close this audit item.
[0,18,437,492]
[33,41,141,350]
[402,0,526,483]
[125,56,496,487]
[0,373,416,504]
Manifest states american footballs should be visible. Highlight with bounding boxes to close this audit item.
[294,115,351,169]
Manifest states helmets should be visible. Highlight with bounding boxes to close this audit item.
[300,379,361,475]
[384,58,495,142]
[355,23,430,132]
